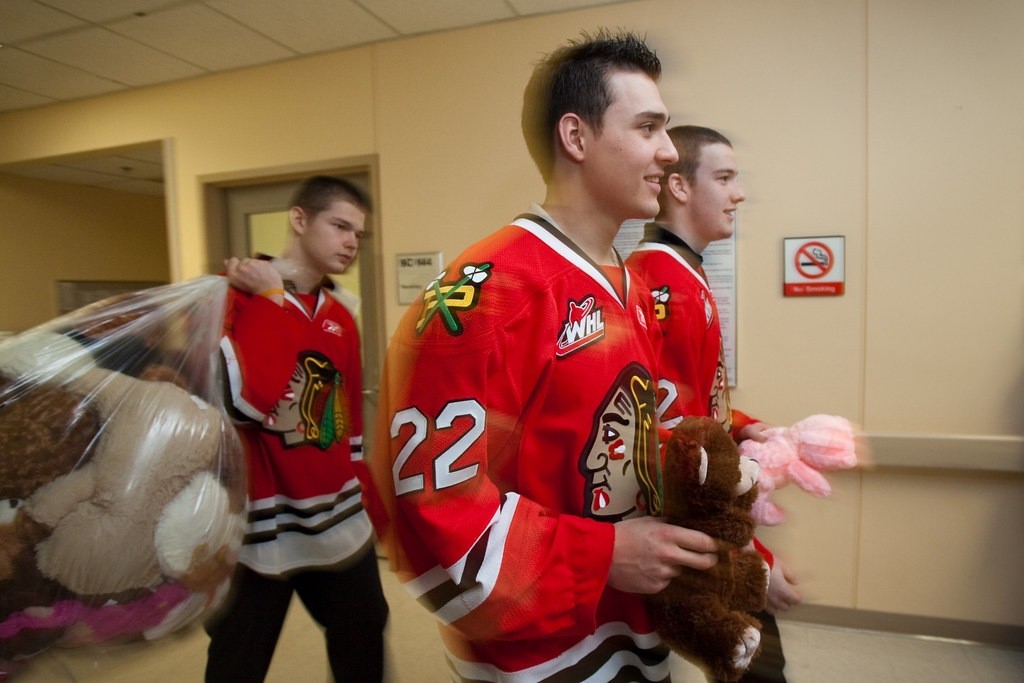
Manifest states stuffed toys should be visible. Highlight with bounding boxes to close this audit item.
[655,417,771,683]
[739,414,856,526]
[0,316,249,645]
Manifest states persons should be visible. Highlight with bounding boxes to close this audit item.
[625,126,796,681]
[376,31,723,682]
[192,175,390,682]
